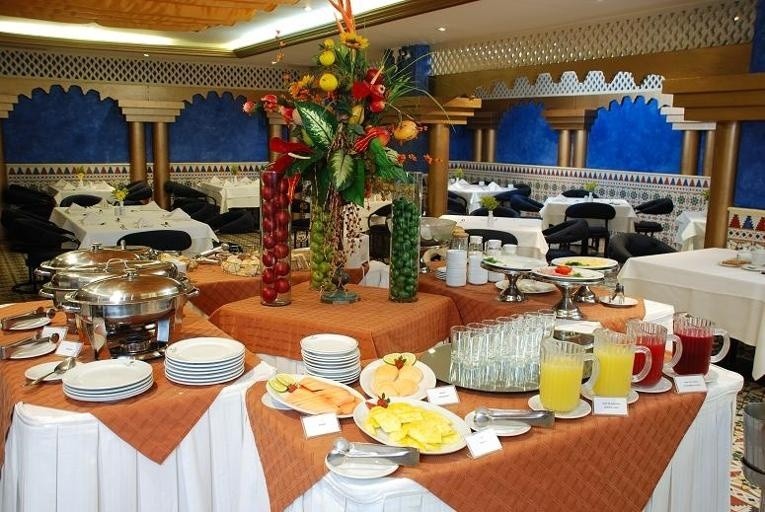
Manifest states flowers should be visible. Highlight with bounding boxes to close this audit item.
[241,0,429,292]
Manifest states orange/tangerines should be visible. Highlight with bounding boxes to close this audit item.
[390,196,420,298]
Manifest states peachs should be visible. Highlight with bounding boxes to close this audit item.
[379,130,391,146]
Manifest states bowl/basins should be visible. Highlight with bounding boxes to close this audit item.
[420,216,455,246]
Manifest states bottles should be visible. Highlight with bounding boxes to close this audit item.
[457,226,517,251]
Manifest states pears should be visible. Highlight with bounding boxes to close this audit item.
[348,105,365,126]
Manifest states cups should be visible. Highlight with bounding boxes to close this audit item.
[446,250,468,287]
[625,322,682,387]
[672,311,731,376]
[470,254,488,286]
[590,326,653,400]
[448,307,556,367]
[538,340,600,415]
[446,365,546,390]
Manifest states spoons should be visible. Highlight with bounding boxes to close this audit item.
[22,356,76,386]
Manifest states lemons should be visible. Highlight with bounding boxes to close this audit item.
[319,50,336,66]
[322,38,334,48]
[320,73,338,92]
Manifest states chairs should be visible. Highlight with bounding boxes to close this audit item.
[1,167,265,294]
[445,179,709,261]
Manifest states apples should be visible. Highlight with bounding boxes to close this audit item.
[310,206,333,288]
[367,69,385,113]
[259,165,290,303]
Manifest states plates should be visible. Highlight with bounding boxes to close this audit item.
[723,255,765,273]
[527,365,718,420]
[436,266,446,281]
[494,281,555,294]
[24,361,82,383]
[61,334,246,403]
[352,396,470,456]
[324,453,397,478]
[466,411,531,437]
[7,317,51,330]
[599,295,639,307]
[299,332,362,384]
[484,254,619,281]
[359,357,437,401]
[10,341,55,358]
[266,374,364,418]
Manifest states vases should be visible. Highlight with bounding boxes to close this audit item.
[320,186,360,304]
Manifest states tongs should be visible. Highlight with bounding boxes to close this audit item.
[1,332,59,359]
[327,436,420,472]
[608,282,626,303]
[193,243,229,266]
[1,306,55,329]
[475,406,558,429]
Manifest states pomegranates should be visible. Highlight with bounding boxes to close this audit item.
[394,120,422,141]
